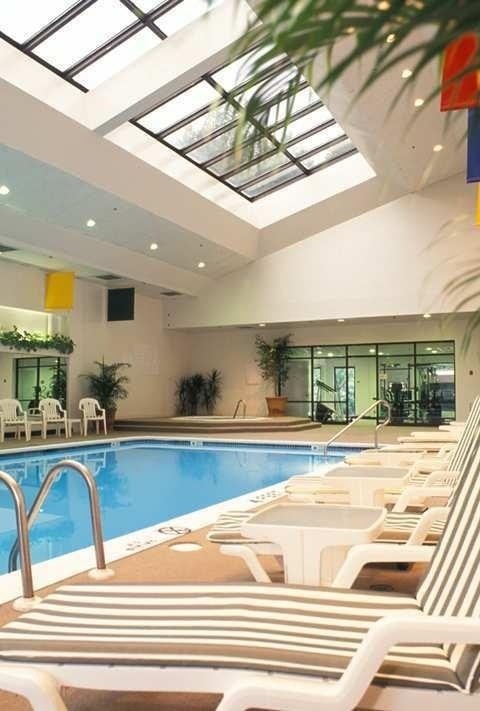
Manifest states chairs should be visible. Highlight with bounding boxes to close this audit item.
[211,393,480,590]
[1,395,111,449]
[0,426,479,711]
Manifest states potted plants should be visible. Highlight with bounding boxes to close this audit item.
[77,353,133,434]
[253,328,297,418]
